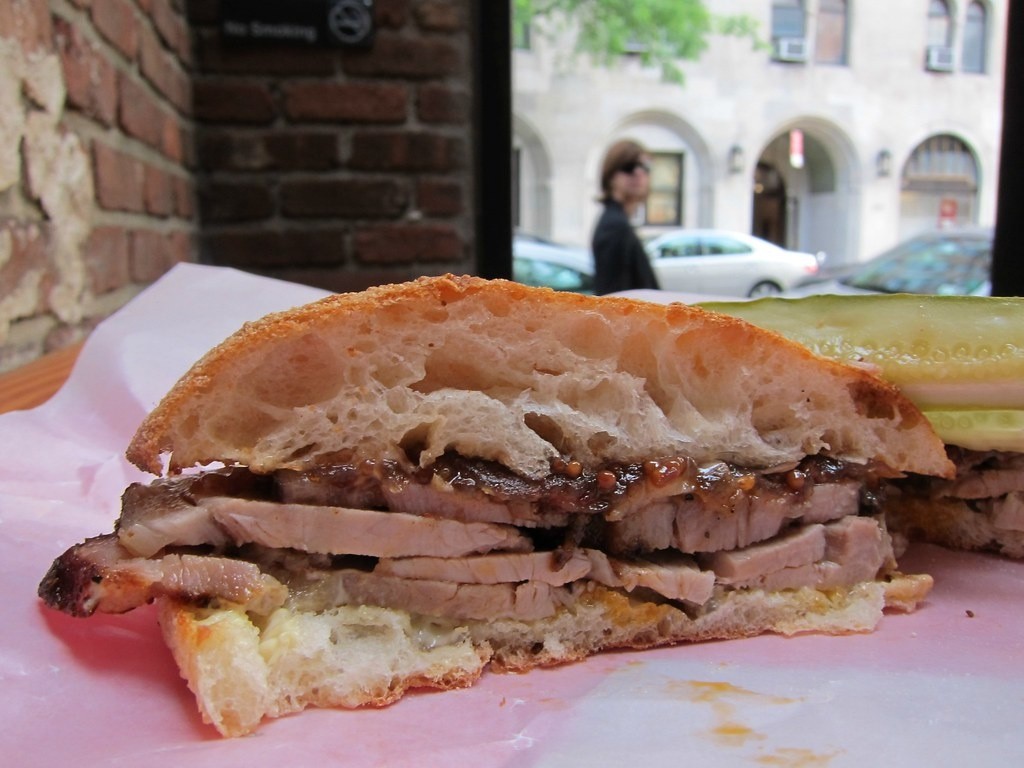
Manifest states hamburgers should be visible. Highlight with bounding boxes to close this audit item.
[35,270,956,747]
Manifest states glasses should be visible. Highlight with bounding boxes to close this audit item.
[637,161,650,174]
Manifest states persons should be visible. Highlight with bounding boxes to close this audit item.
[591,139,666,298]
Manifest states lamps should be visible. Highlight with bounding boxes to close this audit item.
[876,150,892,177]
[729,146,745,173]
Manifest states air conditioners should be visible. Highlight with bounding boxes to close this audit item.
[927,47,958,73]
[771,37,810,63]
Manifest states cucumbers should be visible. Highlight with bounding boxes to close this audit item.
[681,290,1024,453]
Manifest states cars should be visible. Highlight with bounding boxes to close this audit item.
[770,225,995,299]
[512,229,605,299]
[639,226,830,298]
